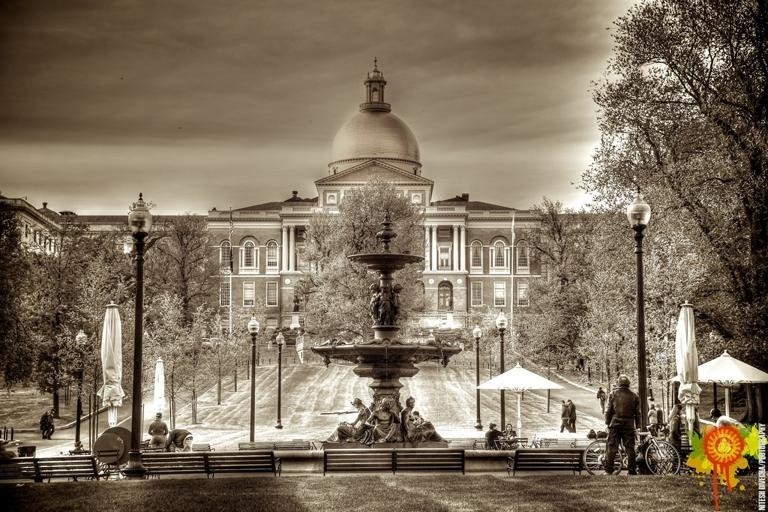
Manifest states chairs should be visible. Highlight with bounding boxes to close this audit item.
[484,436,527,449]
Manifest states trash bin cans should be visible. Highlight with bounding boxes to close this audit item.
[17,445,36,459]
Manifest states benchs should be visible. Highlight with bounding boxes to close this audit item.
[236,440,310,452]
[323,447,465,475]
[189,443,211,452]
[1,457,108,483]
[141,451,281,480]
[539,436,600,448]
[506,449,583,475]
[444,436,486,450]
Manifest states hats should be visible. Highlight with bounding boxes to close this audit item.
[617,375,630,385]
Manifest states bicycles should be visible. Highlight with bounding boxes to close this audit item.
[583,422,682,477]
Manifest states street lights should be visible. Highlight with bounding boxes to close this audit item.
[472,323,485,428]
[274,331,286,429]
[495,310,509,433]
[626,184,653,472]
[246,313,260,440]
[123,192,156,478]
[603,329,651,398]
[71,327,90,454]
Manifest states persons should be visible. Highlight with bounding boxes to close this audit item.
[502,423,519,451]
[39,410,49,438]
[163,428,192,451]
[603,374,640,475]
[0,439,21,486]
[557,385,727,434]
[47,408,57,439]
[147,412,168,448]
[484,420,504,450]
[335,394,446,442]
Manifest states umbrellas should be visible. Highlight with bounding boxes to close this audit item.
[674,300,703,450]
[477,362,566,450]
[153,356,167,416]
[96,300,127,427]
[666,349,768,418]
